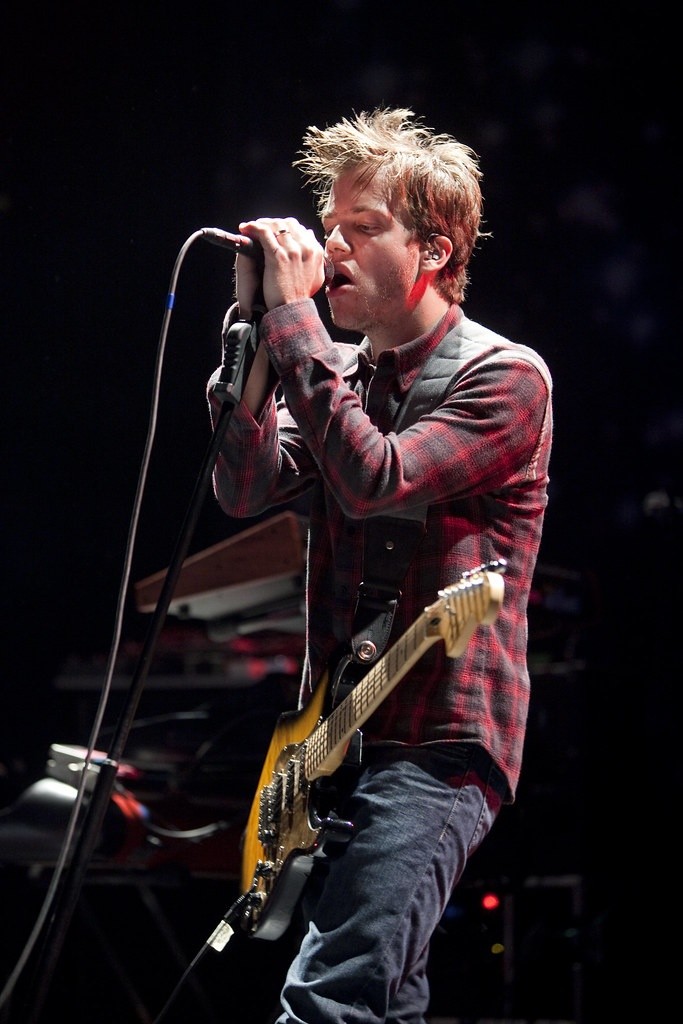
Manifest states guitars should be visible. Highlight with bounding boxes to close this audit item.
[236,553,514,944]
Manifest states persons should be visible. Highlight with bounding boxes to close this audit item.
[206,104,553,1024]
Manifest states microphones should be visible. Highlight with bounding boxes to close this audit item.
[200,228,333,287]
